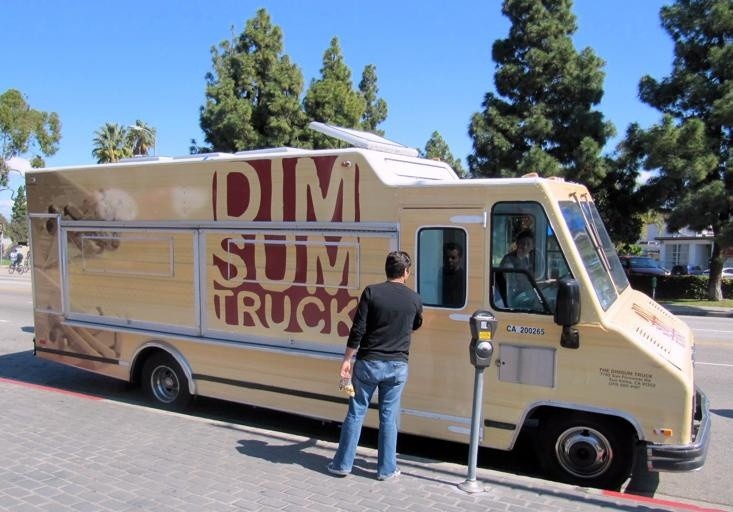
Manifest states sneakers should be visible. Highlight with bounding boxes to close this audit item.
[327,461,349,477]
[377,465,401,481]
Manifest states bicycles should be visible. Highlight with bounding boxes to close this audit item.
[7,262,24,275]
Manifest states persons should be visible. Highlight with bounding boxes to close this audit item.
[497,229,558,311]
[10,249,31,268]
[325,249,424,482]
[435,242,465,308]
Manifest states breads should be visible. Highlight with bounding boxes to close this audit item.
[344,383,355,398]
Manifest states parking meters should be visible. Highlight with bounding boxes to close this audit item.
[453,304,503,496]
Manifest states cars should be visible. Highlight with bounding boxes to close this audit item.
[617,253,672,280]
[670,263,703,277]
[702,266,733,279]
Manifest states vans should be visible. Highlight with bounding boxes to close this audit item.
[20,118,713,486]
